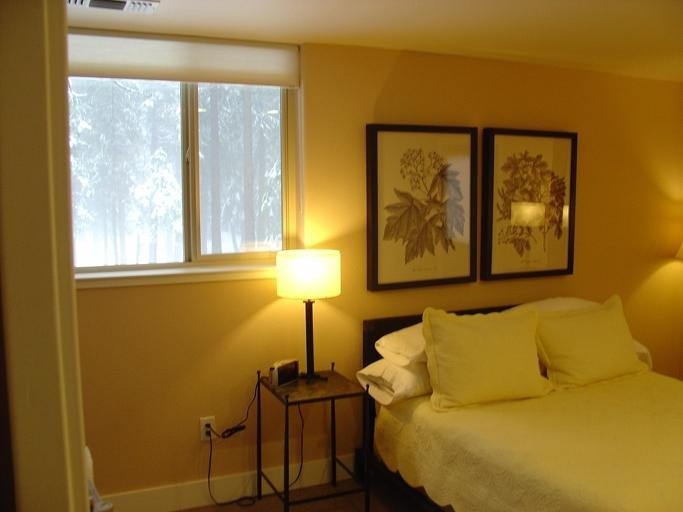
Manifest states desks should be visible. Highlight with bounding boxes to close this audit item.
[257,362,369,512]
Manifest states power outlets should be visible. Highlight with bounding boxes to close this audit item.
[199,416,215,441]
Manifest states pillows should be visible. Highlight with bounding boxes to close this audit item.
[356,294,653,413]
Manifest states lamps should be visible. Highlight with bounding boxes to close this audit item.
[276,249,342,385]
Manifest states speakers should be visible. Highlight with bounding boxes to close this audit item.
[270,358,299,386]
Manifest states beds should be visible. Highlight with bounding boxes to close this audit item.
[363,304,683,512]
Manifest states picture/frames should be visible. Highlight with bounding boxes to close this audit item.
[366,124,477,291]
[480,127,577,280]
